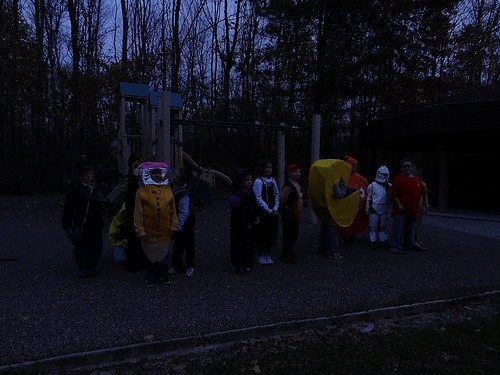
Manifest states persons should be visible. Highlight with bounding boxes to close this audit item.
[109,167,195,284]
[253,160,282,264]
[365,164,393,247]
[343,156,369,246]
[318,181,348,258]
[226,170,264,276]
[410,163,429,245]
[60,166,108,279]
[278,164,309,264]
[390,159,427,254]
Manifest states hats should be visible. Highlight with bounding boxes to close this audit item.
[378,165,390,175]
[345,156,358,166]
[288,163,298,173]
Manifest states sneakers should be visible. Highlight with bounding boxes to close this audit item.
[144,276,155,287]
[157,274,172,284]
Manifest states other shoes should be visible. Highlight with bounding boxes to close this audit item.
[257,255,266,264]
[337,252,343,259]
[283,256,296,264]
[398,247,406,254]
[415,244,428,251]
[167,267,180,274]
[327,252,339,259]
[292,250,298,257]
[265,255,273,265]
[186,266,195,277]
[238,262,251,273]
